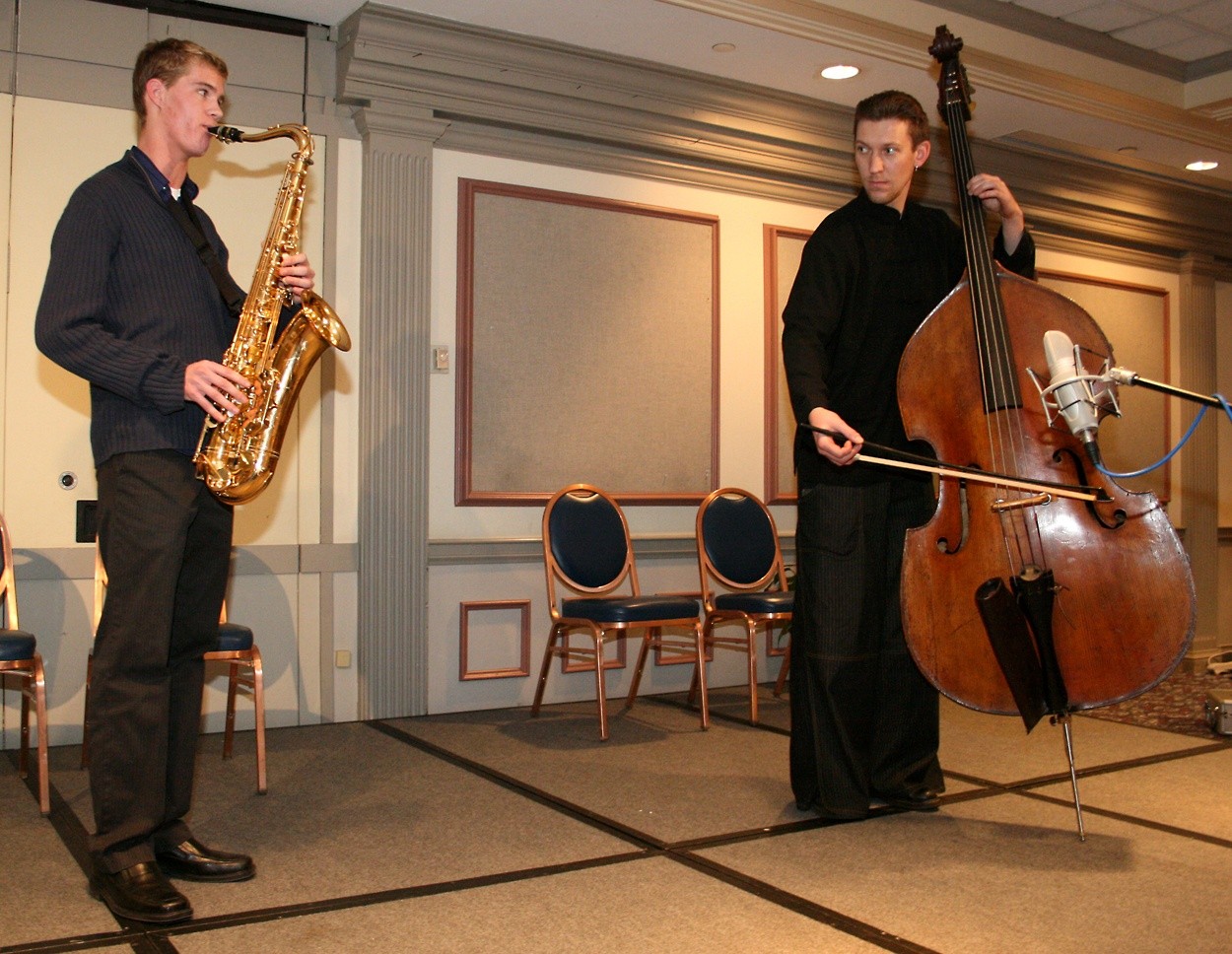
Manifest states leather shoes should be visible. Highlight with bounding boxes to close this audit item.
[158,839,256,885]
[883,785,941,811]
[88,858,195,929]
[794,792,869,820]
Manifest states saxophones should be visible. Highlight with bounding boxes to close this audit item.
[192,121,351,506]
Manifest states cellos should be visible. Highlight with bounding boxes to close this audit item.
[895,24,1199,841]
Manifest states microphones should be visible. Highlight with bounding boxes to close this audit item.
[1043,330,1102,466]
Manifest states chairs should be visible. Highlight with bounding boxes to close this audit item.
[82,530,268,793]
[687,487,795,725]
[0,520,51,813]
[532,483,711,741]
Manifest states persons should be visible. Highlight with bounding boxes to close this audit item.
[781,91,1038,821]
[34,36,316,926]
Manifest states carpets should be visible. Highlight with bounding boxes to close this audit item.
[1072,671,1232,741]
